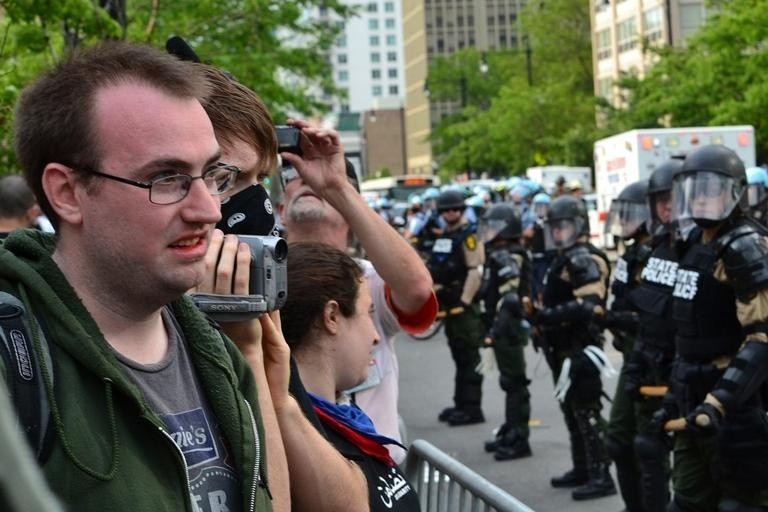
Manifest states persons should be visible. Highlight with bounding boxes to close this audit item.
[1,174,38,236]
[172,50,371,510]
[0,39,274,512]
[275,112,440,470]
[278,234,424,512]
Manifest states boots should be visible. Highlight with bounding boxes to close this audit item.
[612,458,643,512]
[638,459,670,512]
[483,386,533,460]
[570,404,618,501]
[548,397,593,489]
[436,370,485,427]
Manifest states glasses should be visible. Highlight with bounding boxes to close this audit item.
[50,155,246,210]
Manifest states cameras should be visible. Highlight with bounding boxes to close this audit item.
[192,233,289,321]
[274,125,298,154]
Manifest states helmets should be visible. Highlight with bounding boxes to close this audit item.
[646,162,681,196]
[542,195,591,237]
[610,181,652,206]
[479,204,524,240]
[673,142,749,186]
[434,189,467,214]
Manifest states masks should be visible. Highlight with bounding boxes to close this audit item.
[213,181,279,242]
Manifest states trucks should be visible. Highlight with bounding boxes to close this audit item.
[278,132,365,205]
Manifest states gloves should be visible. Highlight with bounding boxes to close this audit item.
[622,371,657,404]
[683,401,725,445]
[650,403,680,434]
[510,298,544,327]
[589,304,616,329]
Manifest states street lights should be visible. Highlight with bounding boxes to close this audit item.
[368,104,407,176]
[476,45,534,88]
[423,75,471,180]
